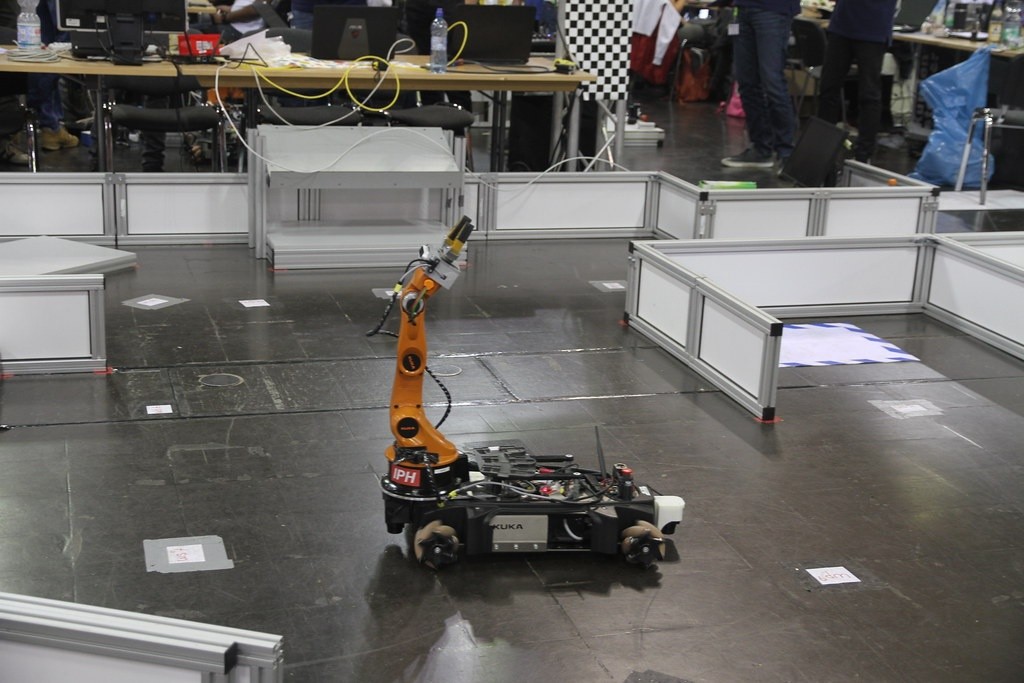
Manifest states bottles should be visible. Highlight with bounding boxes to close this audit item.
[1000,0,1023,49]
[987,0,1003,45]
[945,0,954,30]
[430,8,448,75]
[16,0,42,53]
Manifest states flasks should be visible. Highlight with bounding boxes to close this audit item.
[954,3,968,32]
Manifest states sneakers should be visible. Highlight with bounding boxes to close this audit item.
[777,158,790,176]
[42,127,79,151]
[0,139,29,164]
[721,147,774,167]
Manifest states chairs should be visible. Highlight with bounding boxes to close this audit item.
[238,29,360,172]
[0,27,38,172]
[104,29,228,172]
[362,32,474,170]
[789,16,856,127]
[954,54,1024,205]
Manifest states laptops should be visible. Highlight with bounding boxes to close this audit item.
[447,4,537,65]
[310,5,398,61]
[891,0,940,31]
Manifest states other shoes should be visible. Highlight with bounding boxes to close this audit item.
[849,145,874,163]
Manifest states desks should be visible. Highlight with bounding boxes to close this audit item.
[0,45,595,173]
[795,16,1024,156]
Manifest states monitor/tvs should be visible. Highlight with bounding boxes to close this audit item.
[788,116,848,188]
[56,0,189,65]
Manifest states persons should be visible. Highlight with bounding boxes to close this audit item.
[0,0,894,169]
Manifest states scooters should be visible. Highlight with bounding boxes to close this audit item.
[358,215,686,569]
